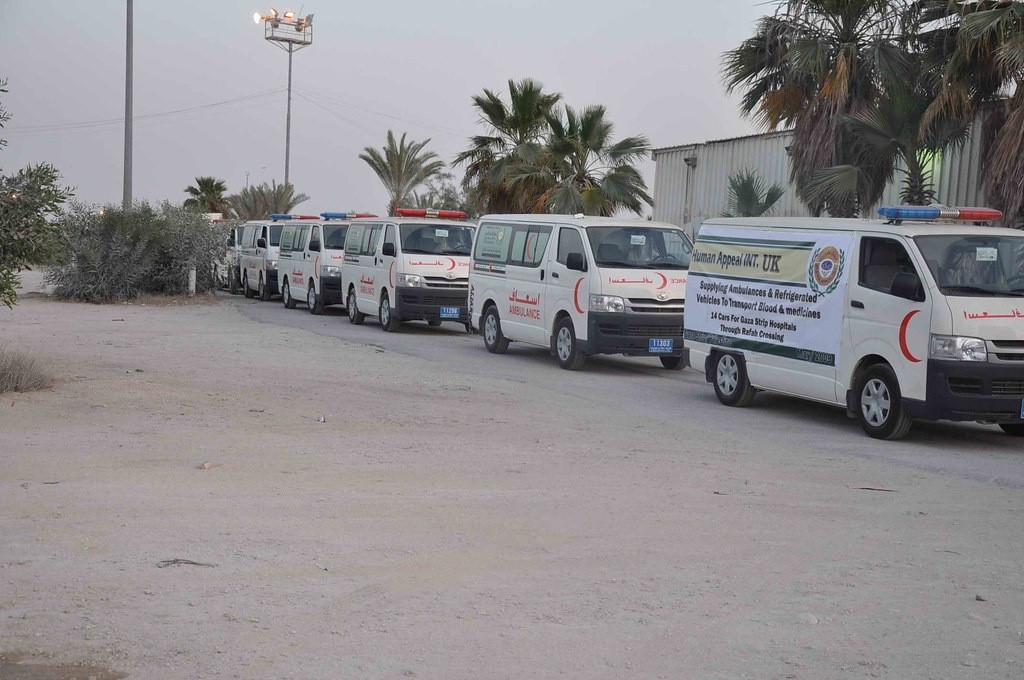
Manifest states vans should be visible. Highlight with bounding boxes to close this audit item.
[277,212,381,315]
[465,213,701,371]
[339,207,481,332]
[237,212,322,302]
[684,199,1024,439]
[210,223,246,295]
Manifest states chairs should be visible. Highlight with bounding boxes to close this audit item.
[863,239,940,297]
[944,247,1012,292]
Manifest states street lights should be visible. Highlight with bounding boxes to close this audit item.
[253,7,316,189]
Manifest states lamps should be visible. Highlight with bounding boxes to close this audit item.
[271,7,279,16]
[684,158,698,167]
[297,17,305,22]
[254,12,261,23]
[784,146,795,156]
[284,11,295,18]
[306,13,315,23]
